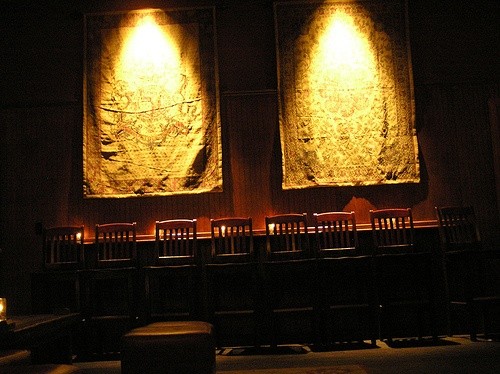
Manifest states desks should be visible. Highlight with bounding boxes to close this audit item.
[0,313,82,365]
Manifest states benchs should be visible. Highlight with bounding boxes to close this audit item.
[120,320,216,374]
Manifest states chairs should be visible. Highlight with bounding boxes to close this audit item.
[262,213,317,306]
[212,272,259,346]
[368,208,434,301]
[269,269,320,352]
[434,204,500,298]
[149,273,189,321]
[30,224,88,313]
[322,266,376,349]
[88,221,137,313]
[143,217,200,312]
[90,279,131,358]
[204,217,259,310]
[311,211,373,304]
[446,261,500,341]
[377,260,438,346]
